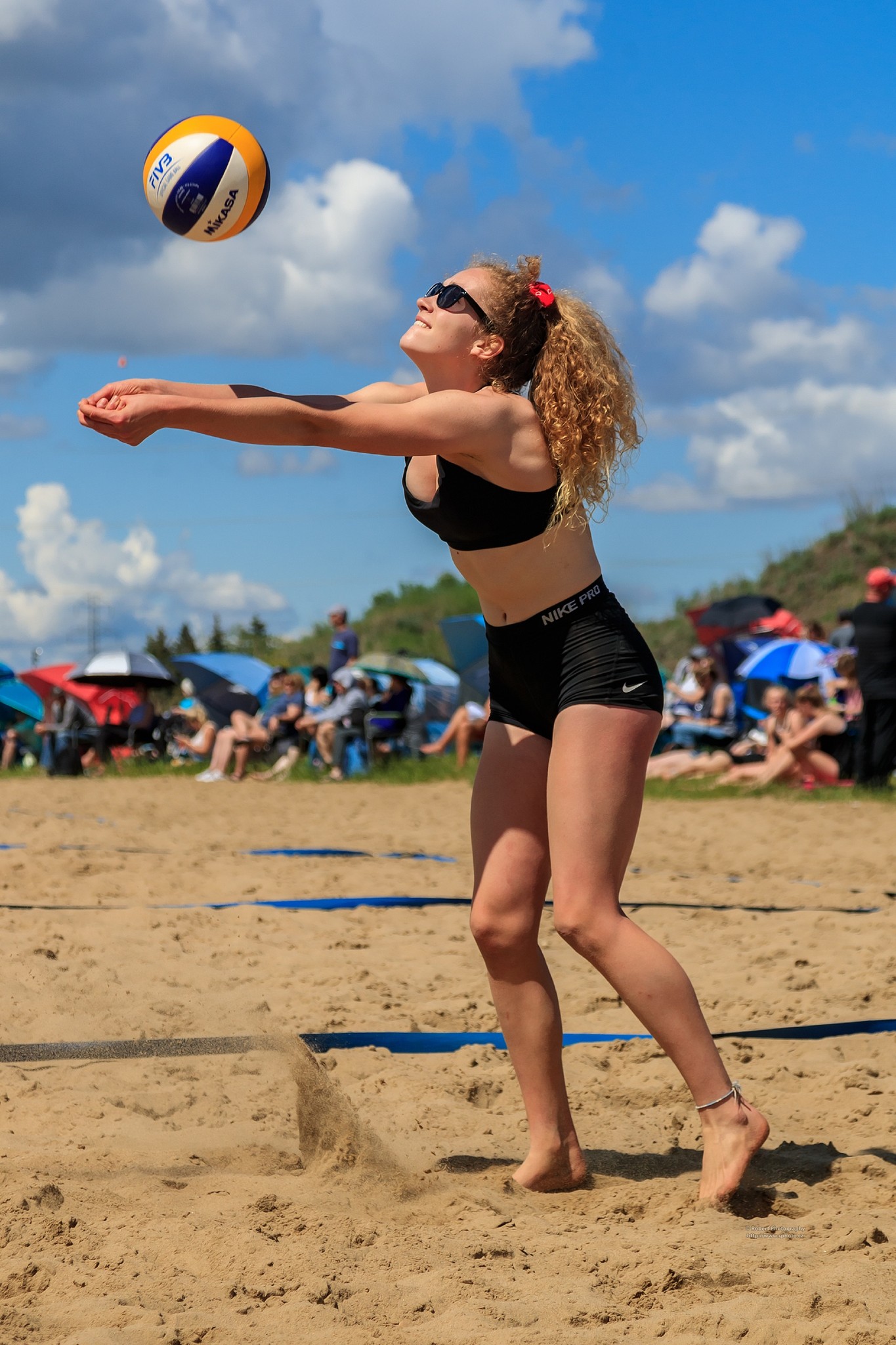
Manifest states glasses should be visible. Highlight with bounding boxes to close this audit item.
[423,281,499,336]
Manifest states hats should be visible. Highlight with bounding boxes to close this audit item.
[865,566,896,586]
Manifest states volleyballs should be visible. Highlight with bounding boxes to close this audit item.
[142,114,271,242]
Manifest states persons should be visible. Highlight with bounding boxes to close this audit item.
[812,565,896,791]
[0,644,866,786]
[76,254,769,1215]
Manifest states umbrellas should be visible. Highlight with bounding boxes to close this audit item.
[0,594,848,744]
